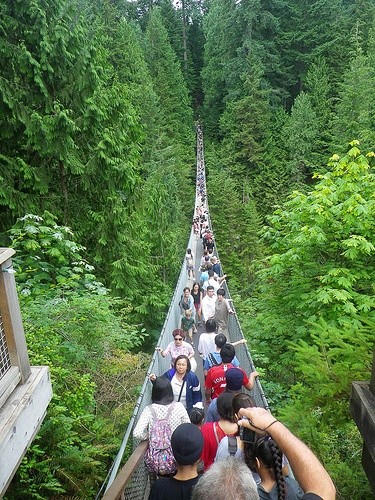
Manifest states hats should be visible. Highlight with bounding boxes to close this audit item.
[171,423,204,465]
[225,368,243,386]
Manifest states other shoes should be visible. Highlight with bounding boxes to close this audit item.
[193,278,195,281]
[198,322,202,327]
[188,277,190,279]
[191,343,196,349]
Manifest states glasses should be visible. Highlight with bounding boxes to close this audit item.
[174,338,183,341]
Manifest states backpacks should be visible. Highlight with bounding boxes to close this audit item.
[144,402,179,475]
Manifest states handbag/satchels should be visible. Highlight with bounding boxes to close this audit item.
[188,254,195,267]
[194,330,197,334]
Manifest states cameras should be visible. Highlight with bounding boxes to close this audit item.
[239,416,257,444]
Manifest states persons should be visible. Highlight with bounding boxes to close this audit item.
[192,406,337,500]
[253,435,305,500]
[147,423,204,500]
[133,376,194,489]
[198,391,238,475]
[148,123,261,425]
[216,393,290,489]
